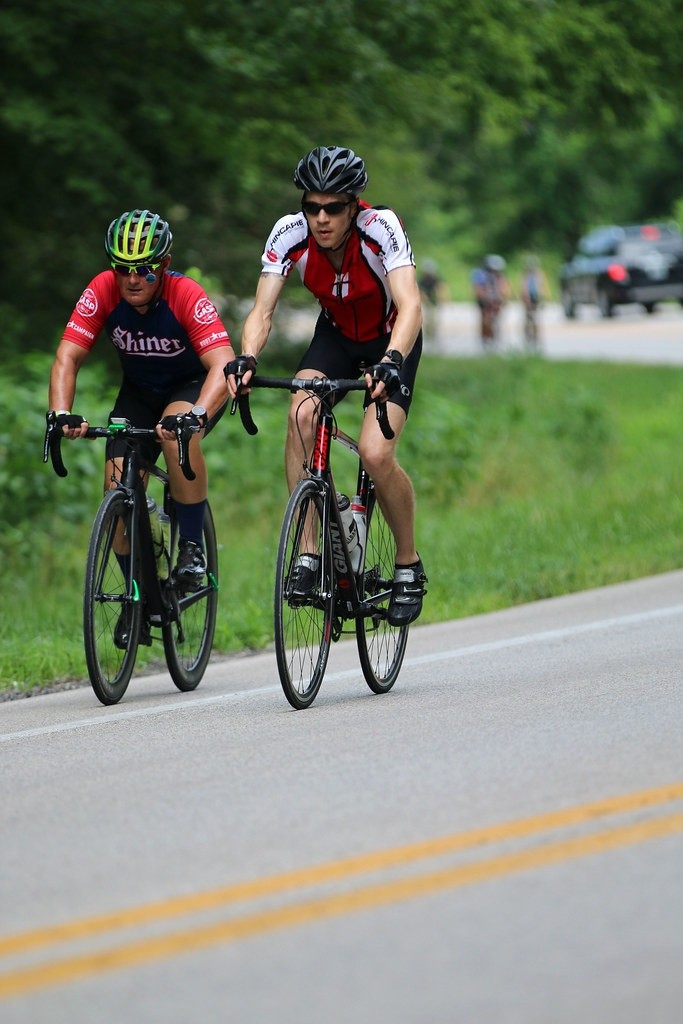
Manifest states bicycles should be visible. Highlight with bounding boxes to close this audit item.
[228,356,410,711]
[40,409,221,706]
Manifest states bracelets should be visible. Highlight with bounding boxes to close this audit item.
[54,410,71,417]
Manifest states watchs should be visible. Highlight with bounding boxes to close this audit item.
[189,406,208,427]
[384,349,403,370]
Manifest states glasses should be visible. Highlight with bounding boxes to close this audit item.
[303,199,353,215]
[110,262,162,276]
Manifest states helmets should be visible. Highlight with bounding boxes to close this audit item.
[292,145,368,195]
[103,209,173,265]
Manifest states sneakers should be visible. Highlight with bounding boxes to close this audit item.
[387,552,428,627]
[283,553,321,596]
[113,602,145,649]
[171,538,207,591]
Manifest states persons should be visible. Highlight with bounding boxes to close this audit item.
[472,255,508,343]
[48,209,235,650]
[223,145,423,627]
[522,257,545,313]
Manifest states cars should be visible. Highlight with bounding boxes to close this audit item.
[559,219,683,319]
